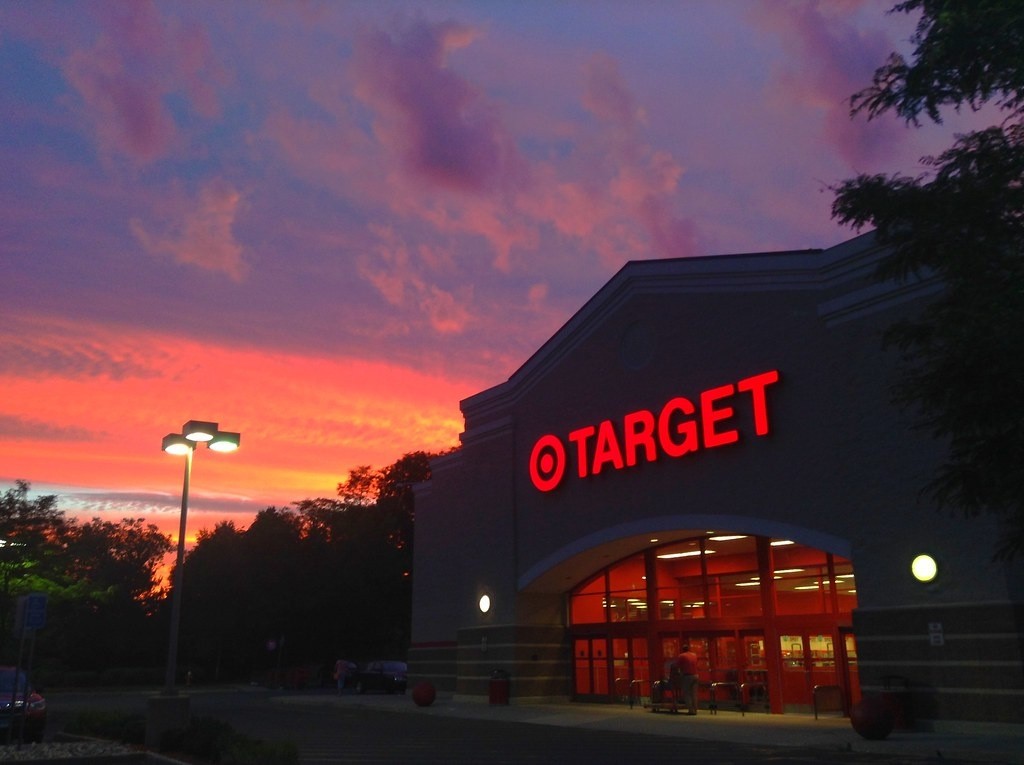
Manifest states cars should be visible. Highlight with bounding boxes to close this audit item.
[334,652,408,694]
[0,665,48,742]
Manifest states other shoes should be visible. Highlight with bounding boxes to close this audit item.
[688,710,696,715]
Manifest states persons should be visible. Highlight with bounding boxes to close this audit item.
[670,644,700,715]
[334,654,351,697]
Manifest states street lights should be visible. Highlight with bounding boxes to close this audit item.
[160,420,242,697]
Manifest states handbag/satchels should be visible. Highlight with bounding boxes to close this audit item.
[336,673,340,680]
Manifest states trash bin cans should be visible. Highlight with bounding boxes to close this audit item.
[880,673,915,733]
[488,677,510,705]
